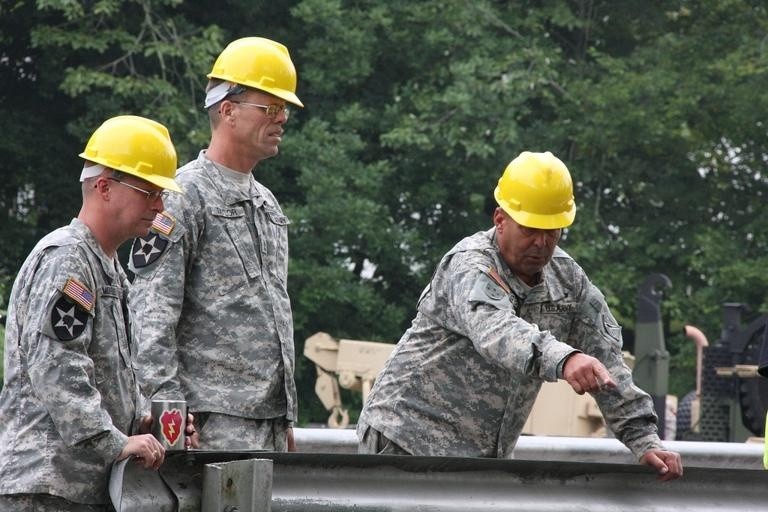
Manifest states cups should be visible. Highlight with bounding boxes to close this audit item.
[151,399,188,450]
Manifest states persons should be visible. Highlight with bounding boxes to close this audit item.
[354,149,685,482]
[124,35,304,456]
[0,113,199,512]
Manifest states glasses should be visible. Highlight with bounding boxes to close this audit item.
[94,176,169,202]
[217,100,290,122]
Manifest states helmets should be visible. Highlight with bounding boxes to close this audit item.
[206,36,304,107]
[494,150,577,230]
[78,114,182,194]
